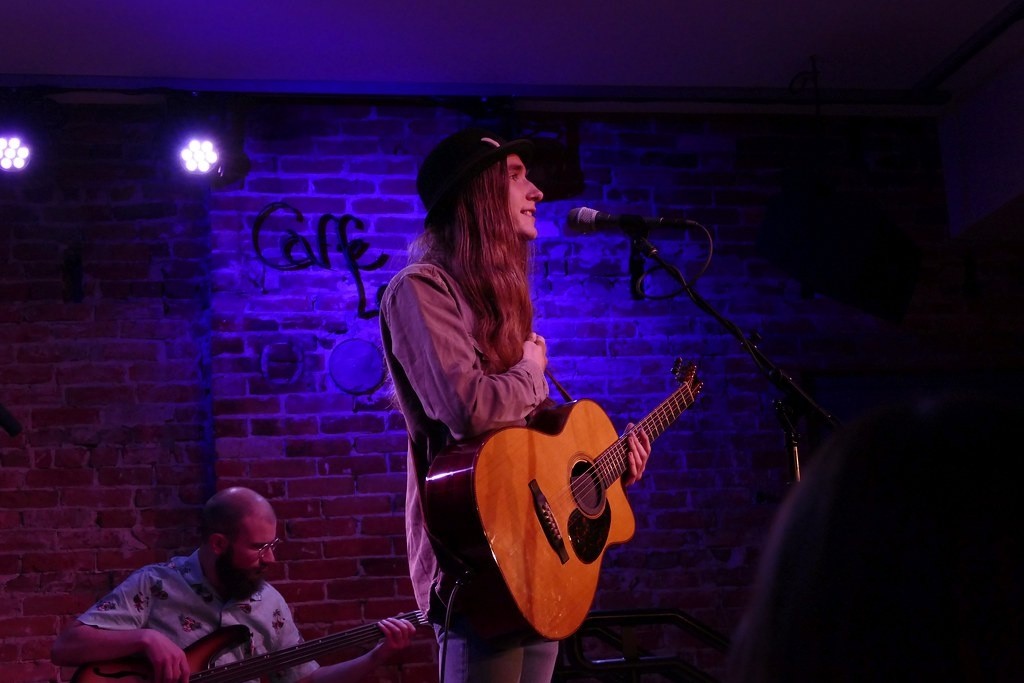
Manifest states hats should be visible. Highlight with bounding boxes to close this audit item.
[417,126,534,231]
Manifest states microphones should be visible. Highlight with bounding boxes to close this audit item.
[568,206,698,233]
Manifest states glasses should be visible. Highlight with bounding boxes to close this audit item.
[226,537,283,558]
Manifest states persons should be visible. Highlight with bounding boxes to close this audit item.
[723,413,1024,683]
[379,126,652,683]
[48,487,415,683]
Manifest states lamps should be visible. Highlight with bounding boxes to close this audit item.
[0,88,44,174]
[174,91,249,189]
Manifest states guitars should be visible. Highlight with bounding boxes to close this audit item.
[68,605,431,682]
[421,357,707,654]
